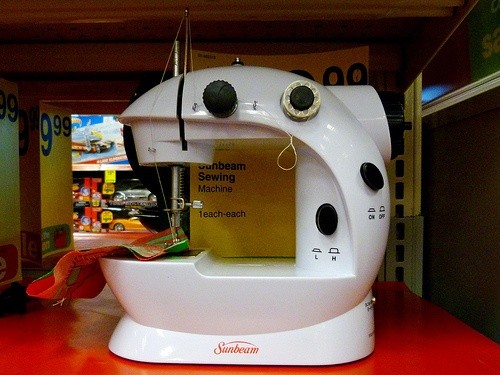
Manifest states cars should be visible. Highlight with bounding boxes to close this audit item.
[111,183,158,206]
[109,213,157,233]
